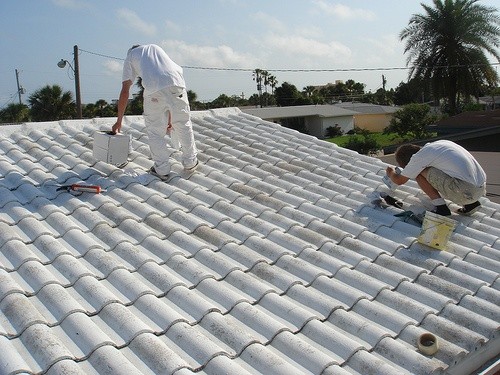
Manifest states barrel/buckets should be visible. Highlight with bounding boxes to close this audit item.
[417,211,458,251]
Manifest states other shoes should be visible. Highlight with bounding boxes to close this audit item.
[185,160,199,172]
[150,166,168,179]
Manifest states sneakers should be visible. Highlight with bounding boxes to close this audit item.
[457,201,481,216]
[422,204,452,218]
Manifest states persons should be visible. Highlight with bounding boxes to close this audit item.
[386,139,487,218]
[112,43,199,179]
